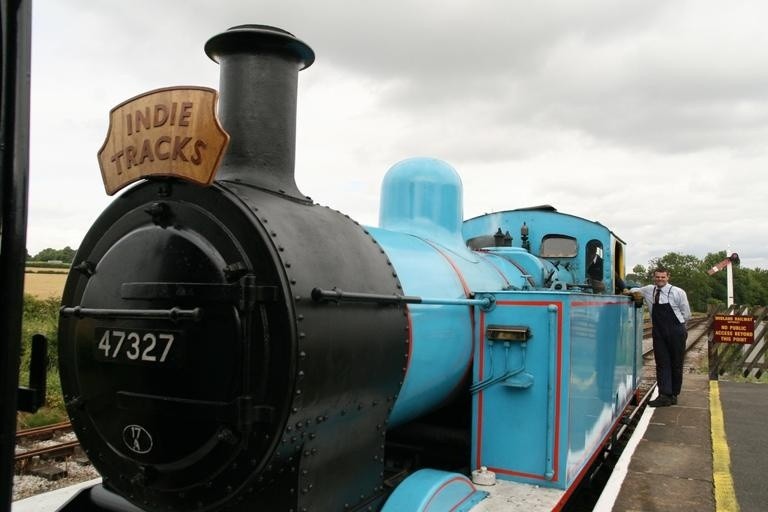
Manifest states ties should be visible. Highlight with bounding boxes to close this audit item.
[655,289,661,304]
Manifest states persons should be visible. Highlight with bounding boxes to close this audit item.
[629,269,691,407]
[615,271,629,294]
[594,298,628,401]
[586,242,605,293]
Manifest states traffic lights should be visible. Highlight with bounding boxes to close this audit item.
[730,252,737,263]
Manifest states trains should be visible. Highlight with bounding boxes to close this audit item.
[54,23,645,511]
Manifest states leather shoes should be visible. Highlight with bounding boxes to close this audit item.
[648,394,676,406]
